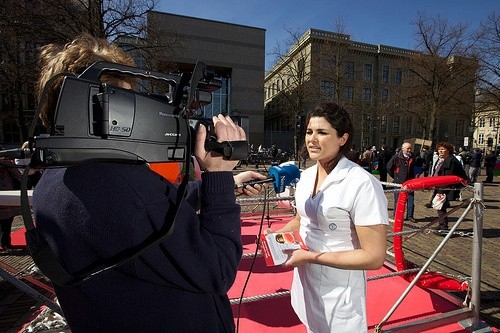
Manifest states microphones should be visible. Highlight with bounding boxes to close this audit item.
[234,162,301,194]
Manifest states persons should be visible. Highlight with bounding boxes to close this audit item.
[0,141,40,256]
[436,143,471,237]
[469,148,481,183]
[255,103,389,333]
[378,144,388,190]
[424,151,451,208]
[482,150,497,183]
[386,142,417,223]
[346,143,483,186]
[247,143,289,170]
[33,34,270,333]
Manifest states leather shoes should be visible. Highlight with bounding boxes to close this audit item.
[407,217,417,223]
[431,225,449,230]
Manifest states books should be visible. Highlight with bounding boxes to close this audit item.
[257,230,310,267]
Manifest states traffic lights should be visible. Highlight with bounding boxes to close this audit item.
[297,114,305,133]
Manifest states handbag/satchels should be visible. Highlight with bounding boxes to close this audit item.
[432,193,446,210]
